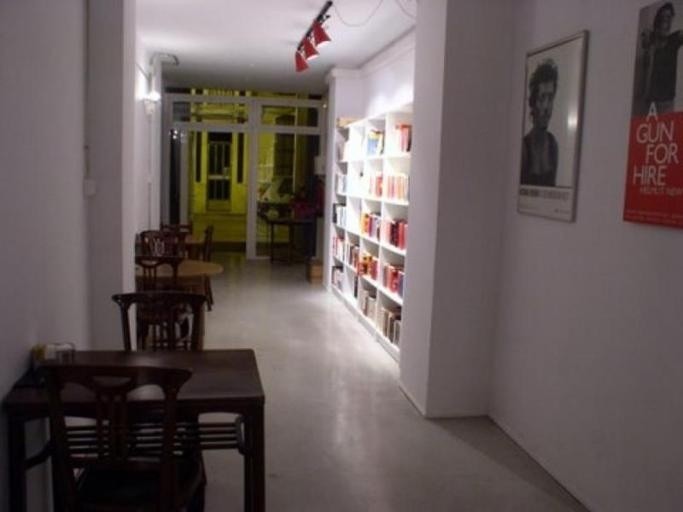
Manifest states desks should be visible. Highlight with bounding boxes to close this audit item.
[134,257,225,311]
[254,210,317,267]
[1,346,268,511]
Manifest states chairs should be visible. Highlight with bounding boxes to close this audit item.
[139,223,194,262]
[110,290,209,351]
[41,362,207,509]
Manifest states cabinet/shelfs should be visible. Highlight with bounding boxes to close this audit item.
[326,100,414,369]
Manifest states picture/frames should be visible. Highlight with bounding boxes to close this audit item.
[515,28,589,224]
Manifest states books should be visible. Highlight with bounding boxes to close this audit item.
[330,125,412,348]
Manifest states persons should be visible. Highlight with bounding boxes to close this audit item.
[521,58,559,186]
[633,2,683,115]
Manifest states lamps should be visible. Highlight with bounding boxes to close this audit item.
[292,23,334,75]
